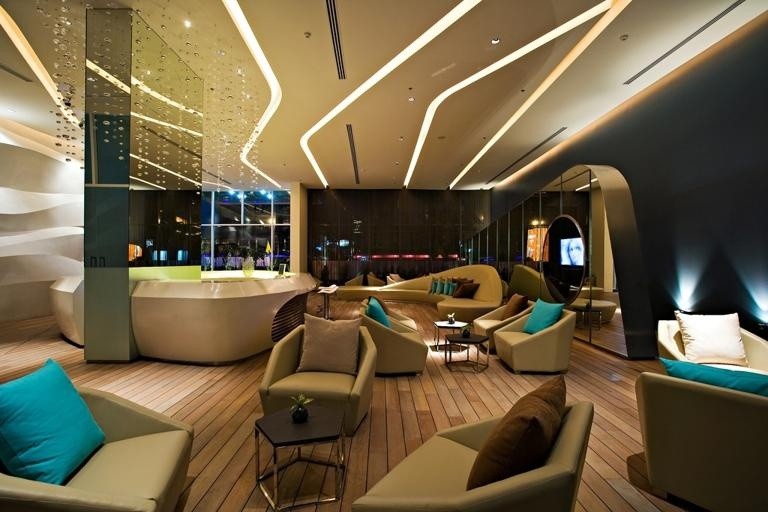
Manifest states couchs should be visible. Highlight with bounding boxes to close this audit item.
[509,263,617,322]
[336,264,504,321]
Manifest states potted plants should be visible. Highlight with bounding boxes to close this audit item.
[287,392,314,423]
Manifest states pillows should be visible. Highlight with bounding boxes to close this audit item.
[295,311,363,375]
[0,358,106,486]
[427,277,480,299]
[466,375,567,491]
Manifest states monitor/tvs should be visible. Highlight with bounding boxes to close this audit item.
[558,233,586,270]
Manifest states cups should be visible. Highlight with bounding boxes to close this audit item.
[243,262,254,277]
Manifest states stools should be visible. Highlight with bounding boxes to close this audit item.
[317,286,339,320]
[318,284,337,317]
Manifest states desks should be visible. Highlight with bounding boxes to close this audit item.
[571,306,603,331]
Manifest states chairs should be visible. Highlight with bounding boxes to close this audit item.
[258,325,378,437]
[350,401,594,512]
[345,272,405,286]
[0,387,193,512]
[634,309,768,512]
[359,293,576,375]
